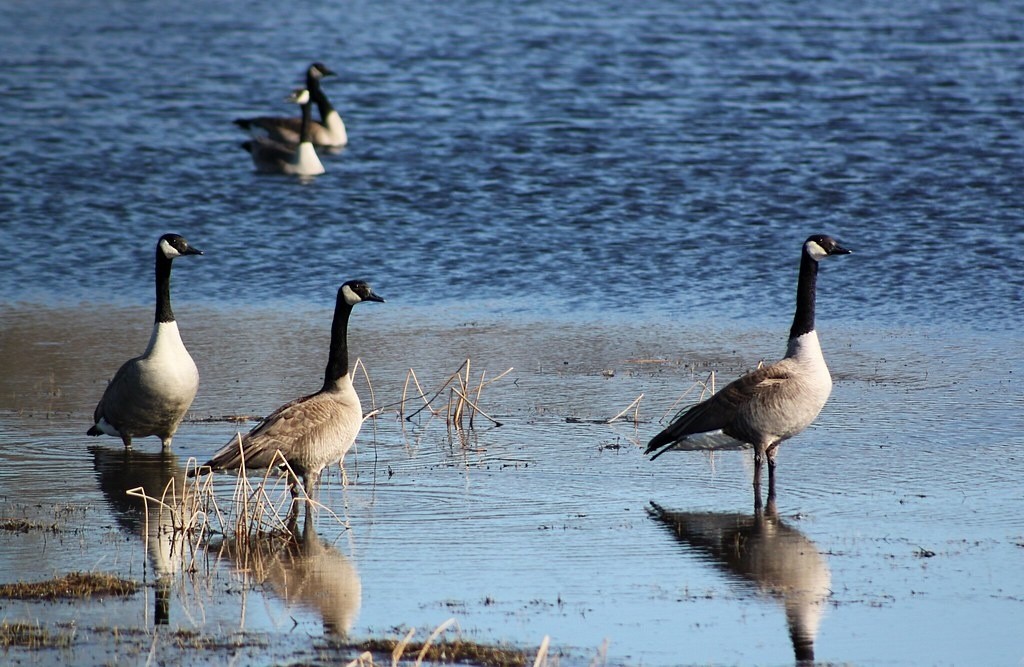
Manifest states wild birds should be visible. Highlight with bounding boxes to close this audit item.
[85,445,202,666]
[187,507,374,667]
[643,499,828,667]
[84,233,205,455]
[643,234,851,531]
[231,62,348,175]
[185,279,387,542]
